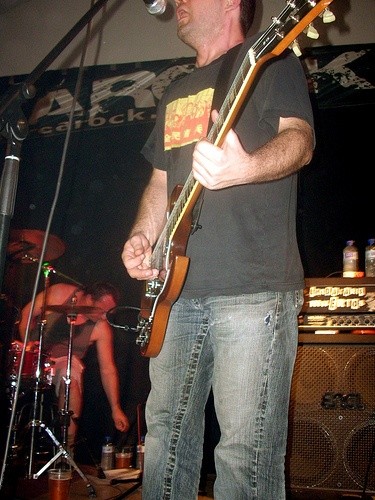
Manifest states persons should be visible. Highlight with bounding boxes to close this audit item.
[121,0,315,500]
[16,280,129,465]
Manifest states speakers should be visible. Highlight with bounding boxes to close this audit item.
[284,333,375,500]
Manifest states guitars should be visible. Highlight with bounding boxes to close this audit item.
[136,0,336,358]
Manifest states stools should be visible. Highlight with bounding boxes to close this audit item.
[7,376,60,462]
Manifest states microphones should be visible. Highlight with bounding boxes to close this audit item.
[141,0,167,16]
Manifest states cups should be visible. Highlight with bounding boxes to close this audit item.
[115,446,133,468]
[48,468,72,500]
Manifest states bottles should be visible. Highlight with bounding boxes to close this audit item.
[364,239,375,277]
[342,240,358,272]
[135,436,145,469]
[100,437,114,471]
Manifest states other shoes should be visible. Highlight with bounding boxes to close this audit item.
[56,449,74,464]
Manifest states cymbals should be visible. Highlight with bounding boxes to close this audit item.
[40,305,106,315]
[7,230,65,263]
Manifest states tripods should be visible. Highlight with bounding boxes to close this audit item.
[13,252,94,496]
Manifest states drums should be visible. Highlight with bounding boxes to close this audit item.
[8,350,53,390]
